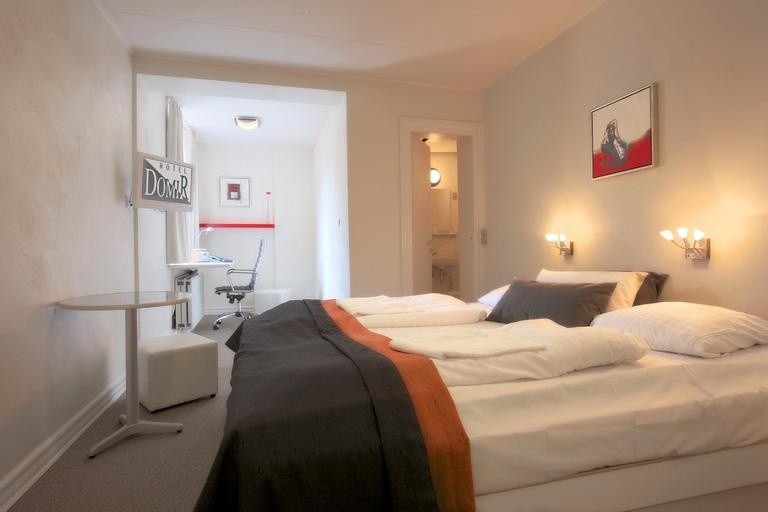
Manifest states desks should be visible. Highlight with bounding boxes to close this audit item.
[57,289,192,460]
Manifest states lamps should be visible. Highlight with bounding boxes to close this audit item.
[546,233,574,257]
[659,226,711,261]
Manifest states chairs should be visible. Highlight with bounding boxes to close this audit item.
[211,238,266,331]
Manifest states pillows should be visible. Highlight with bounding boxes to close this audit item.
[476,266,767,360]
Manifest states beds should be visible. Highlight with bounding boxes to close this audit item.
[229,282,767,511]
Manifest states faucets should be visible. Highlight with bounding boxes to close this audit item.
[432,251,437,256]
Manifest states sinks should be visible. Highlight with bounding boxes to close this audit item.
[432,257,459,269]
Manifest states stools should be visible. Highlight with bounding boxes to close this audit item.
[138,330,219,413]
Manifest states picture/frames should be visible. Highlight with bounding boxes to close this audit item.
[588,81,659,181]
